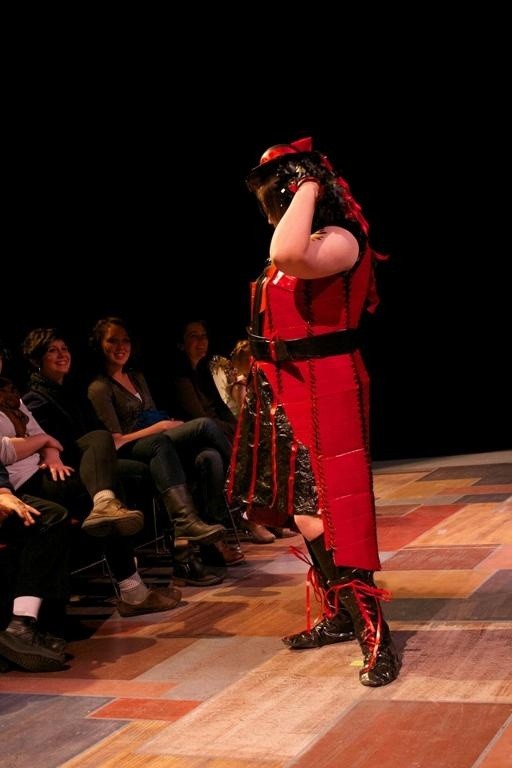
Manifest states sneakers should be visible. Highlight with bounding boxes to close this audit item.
[34,625,80,658]
[1,621,65,670]
[116,590,177,617]
[82,497,142,536]
[241,520,298,544]
[200,542,245,567]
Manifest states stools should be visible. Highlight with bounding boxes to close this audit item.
[115,493,171,579]
[215,485,247,550]
[47,511,125,620]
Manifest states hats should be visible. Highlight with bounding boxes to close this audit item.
[247,143,321,175]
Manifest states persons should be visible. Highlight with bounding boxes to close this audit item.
[0,350,182,617]
[148,316,299,536]
[243,135,402,685]
[0,462,67,672]
[86,316,299,542]
[21,330,227,587]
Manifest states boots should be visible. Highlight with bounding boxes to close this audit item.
[162,483,226,547]
[283,538,357,649]
[163,520,223,585]
[310,531,402,687]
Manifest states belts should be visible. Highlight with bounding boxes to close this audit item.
[253,329,358,363]
